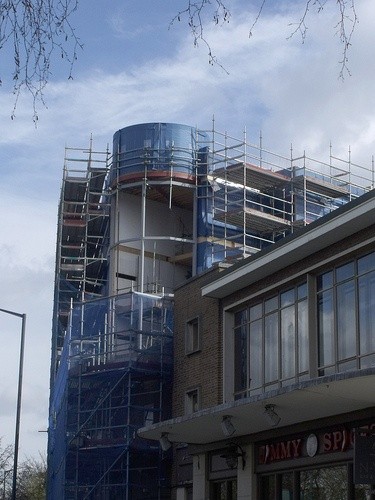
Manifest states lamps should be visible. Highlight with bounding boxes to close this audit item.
[219,443,247,471]
[263,403,280,427]
[160,432,172,450]
[220,415,237,436]
